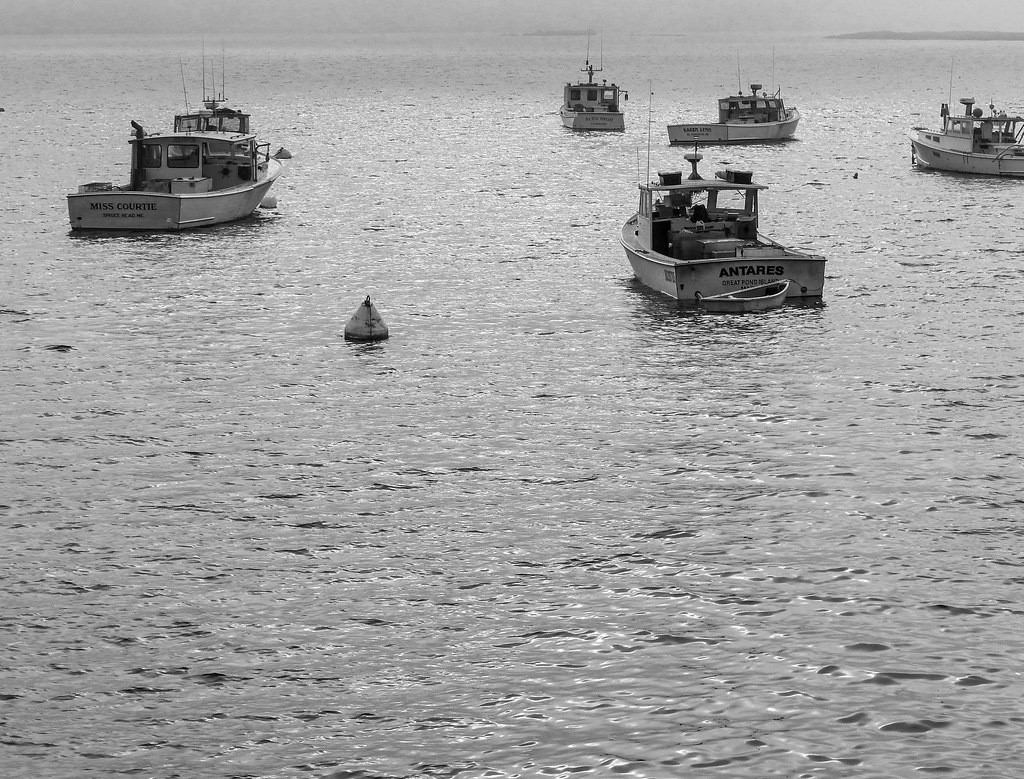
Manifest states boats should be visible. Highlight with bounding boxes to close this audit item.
[665,47,801,146]
[558,26,626,133]
[65,39,291,233]
[908,57,1024,180]
[692,278,791,315]
[619,79,828,306]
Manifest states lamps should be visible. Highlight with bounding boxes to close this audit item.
[619,90,628,101]
